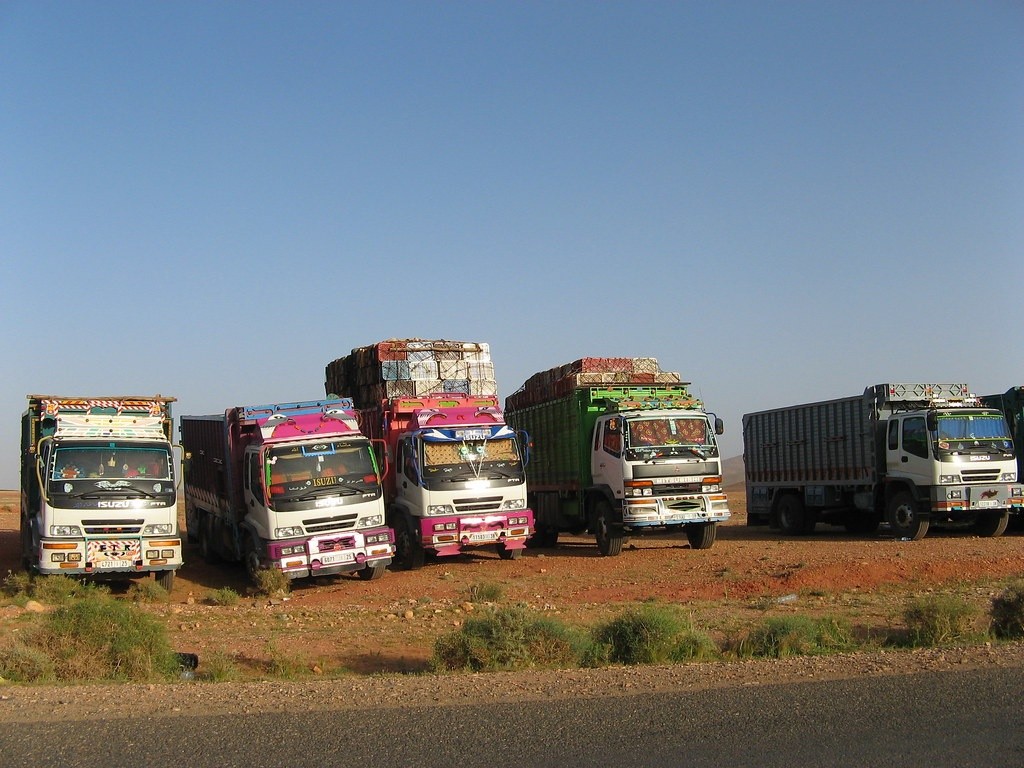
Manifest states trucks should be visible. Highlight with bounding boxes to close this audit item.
[175,398,398,591]
[974,385,1024,485]
[741,382,1023,542]
[500,380,731,557]
[19,394,188,595]
[369,392,537,562]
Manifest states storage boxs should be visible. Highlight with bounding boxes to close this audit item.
[324,337,498,409]
[505,357,680,412]
